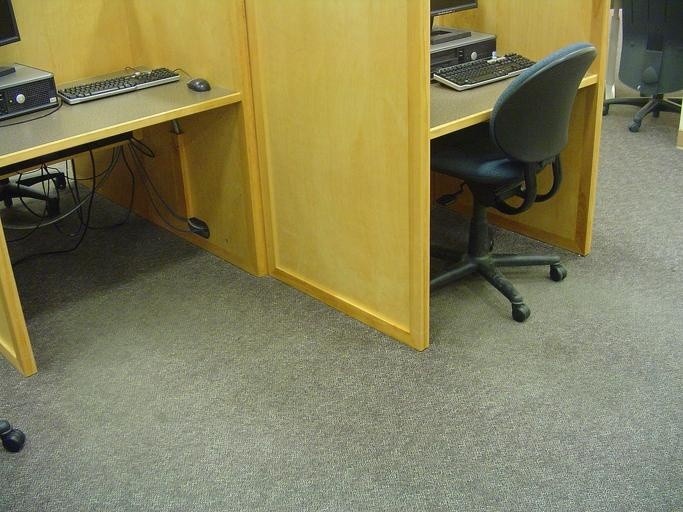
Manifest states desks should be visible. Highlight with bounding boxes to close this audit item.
[1,66,242,378]
[430,58,597,141]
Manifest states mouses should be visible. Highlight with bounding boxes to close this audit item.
[186,78,210,91]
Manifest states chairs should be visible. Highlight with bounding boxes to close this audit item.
[603,2,683,131]
[430,44,598,322]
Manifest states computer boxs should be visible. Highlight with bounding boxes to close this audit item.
[0,63,59,121]
[430,27,497,83]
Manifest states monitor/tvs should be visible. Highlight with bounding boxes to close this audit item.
[430,0,478,45]
[0,0,22,78]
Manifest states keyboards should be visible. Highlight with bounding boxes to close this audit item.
[57,68,181,105]
[433,52,536,92]
